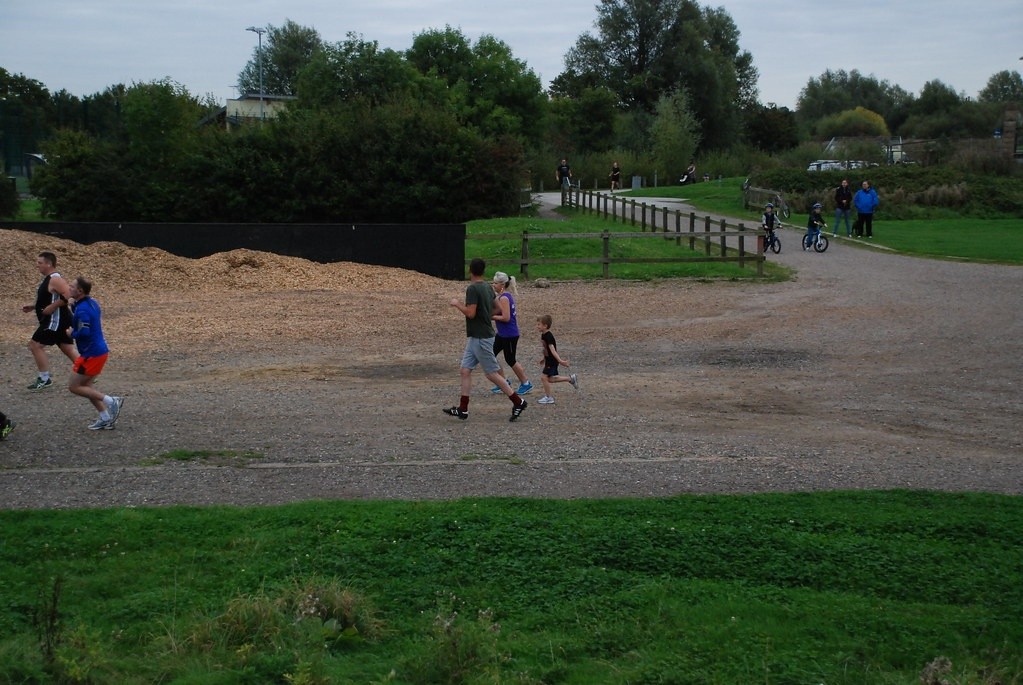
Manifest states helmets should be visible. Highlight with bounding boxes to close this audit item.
[813,203,823,209]
[764,202,774,208]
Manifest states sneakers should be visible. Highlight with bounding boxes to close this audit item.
[516,379,536,394]
[87,418,116,431]
[110,396,125,419]
[537,395,555,404]
[26,377,54,391]
[569,373,579,389]
[508,399,528,422]
[0,419,18,440]
[442,406,469,420]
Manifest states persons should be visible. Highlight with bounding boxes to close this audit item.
[806,202,828,251]
[833,179,853,238]
[22,252,99,392]
[853,180,880,239]
[0,412,17,441]
[608,161,620,192]
[441,257,528,423]
[490,271,534,395]
[65,275,125,431]
[555,158,573,190]
[762,203,782,247]
[536,314,579,404]
[688,162,696,184]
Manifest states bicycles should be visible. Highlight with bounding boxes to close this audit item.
[774,188,790,219]
[802,223,829,253]
[762,226,783,254]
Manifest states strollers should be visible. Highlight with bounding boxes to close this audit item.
[679,170,691,185]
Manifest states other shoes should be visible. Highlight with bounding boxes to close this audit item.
[833,233,837,238]
[869,235,873,239]
[846,234,852,238]
[857,234,862,237]
